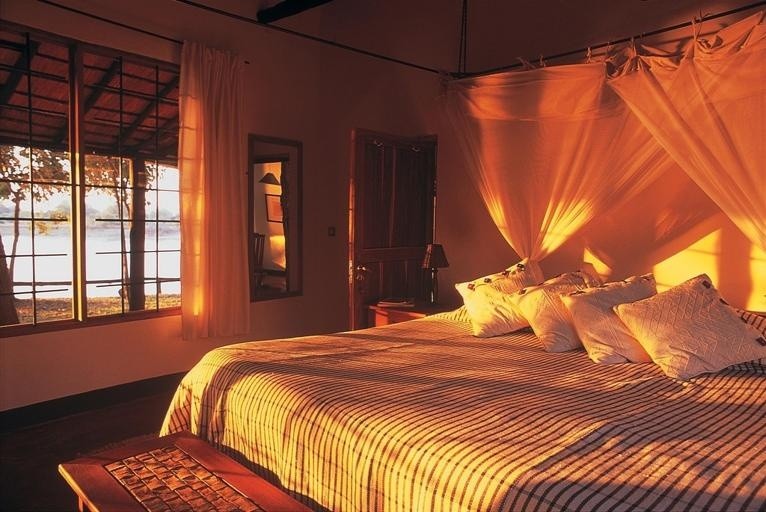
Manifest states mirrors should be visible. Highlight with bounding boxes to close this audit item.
[247,132,303,303]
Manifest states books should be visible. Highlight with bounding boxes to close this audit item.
[378,295,417,308]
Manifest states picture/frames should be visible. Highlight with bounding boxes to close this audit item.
[265,194,284,223]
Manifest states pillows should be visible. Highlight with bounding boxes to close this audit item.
[455,258,547,339]
[614,274,766,380]
[508,270,604,353]
[559,273,658,363]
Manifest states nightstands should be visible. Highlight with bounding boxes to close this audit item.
[368,301,452,327]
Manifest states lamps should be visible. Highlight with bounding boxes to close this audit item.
[421,244,450,305]
[259,172,281,185]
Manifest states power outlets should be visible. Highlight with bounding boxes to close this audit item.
[328,227,336,236]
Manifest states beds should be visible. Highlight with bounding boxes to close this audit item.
[157,311,766,511]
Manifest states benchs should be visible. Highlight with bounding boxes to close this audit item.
[59,434,318,512]
